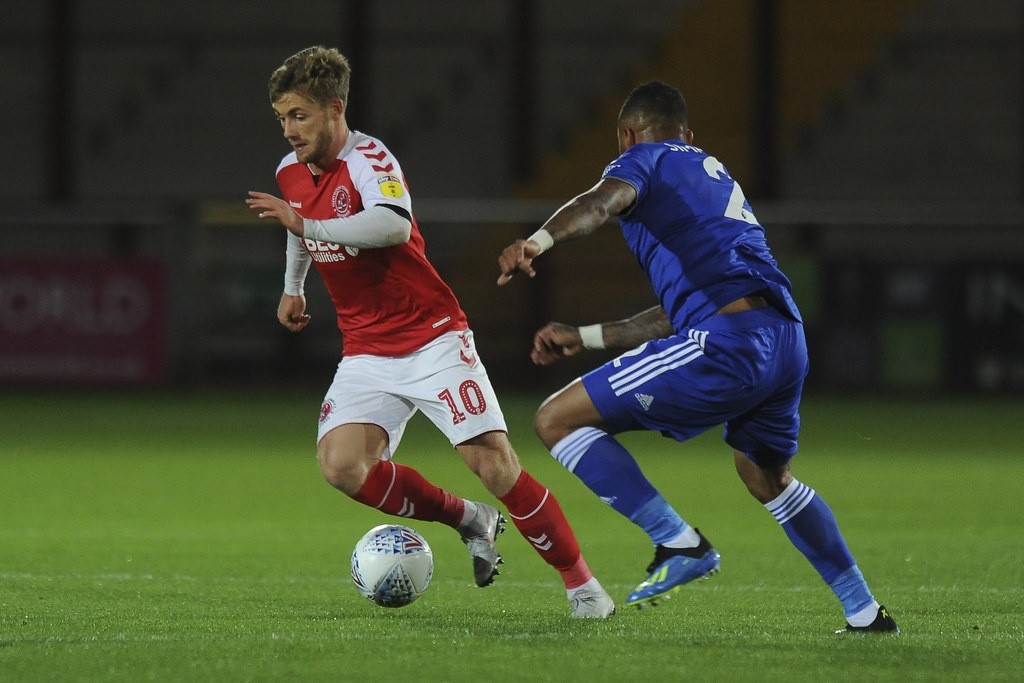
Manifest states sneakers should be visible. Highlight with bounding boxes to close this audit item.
[568,577,616,620]
[833,605,900,637]
[626,528,720,607]
[460,502,508,588]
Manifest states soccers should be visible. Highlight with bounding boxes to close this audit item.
[349,524,434,608]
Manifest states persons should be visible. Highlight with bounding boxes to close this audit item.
[245,43,617,620]
[496,79,901,639]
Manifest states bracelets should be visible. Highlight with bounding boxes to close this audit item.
[577,322,607,350]
[527,227,555,256]
